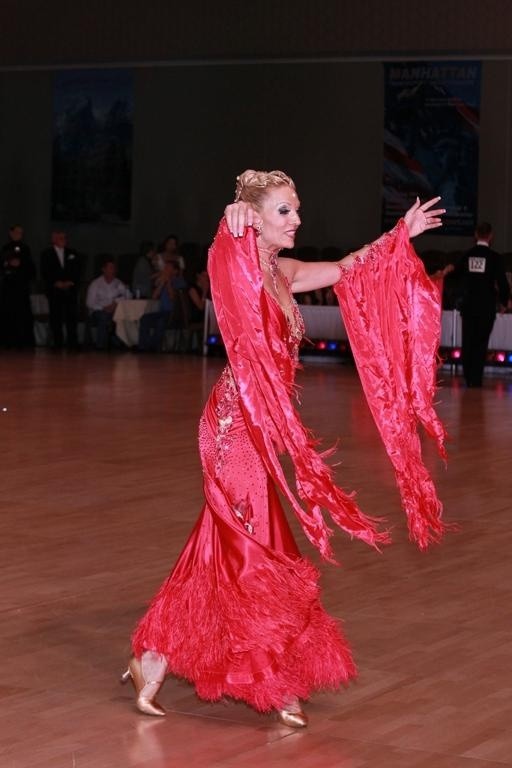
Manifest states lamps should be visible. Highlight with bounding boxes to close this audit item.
[31,285,204,360]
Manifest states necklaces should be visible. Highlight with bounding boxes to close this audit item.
[256,247,288,297]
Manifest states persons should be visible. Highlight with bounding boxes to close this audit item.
[303,289,334,306]
[131,236,211,355]
[119,169,462,727]
[40,229,84,355]
[444,222,511,388]
[85,261,132,351]
[2,223,36,350]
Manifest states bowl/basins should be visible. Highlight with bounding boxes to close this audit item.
[256,247,288,297]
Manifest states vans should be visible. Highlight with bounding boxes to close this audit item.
[120,653,166,717]
[274,708,309,728]
